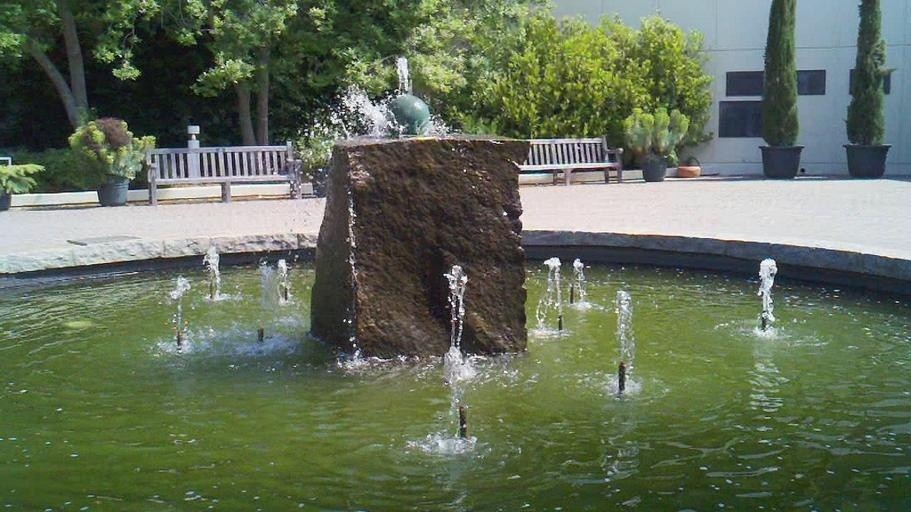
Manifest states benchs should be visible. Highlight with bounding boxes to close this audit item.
[145,141,303,207]
[517,135,624,186]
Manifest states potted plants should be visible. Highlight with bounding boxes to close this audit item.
[297,136,333,198]
[68,117,156,207]
[841,0,894,180]
[0,164,46,212]
[621,108,689,182]
[757,0,806,180]
